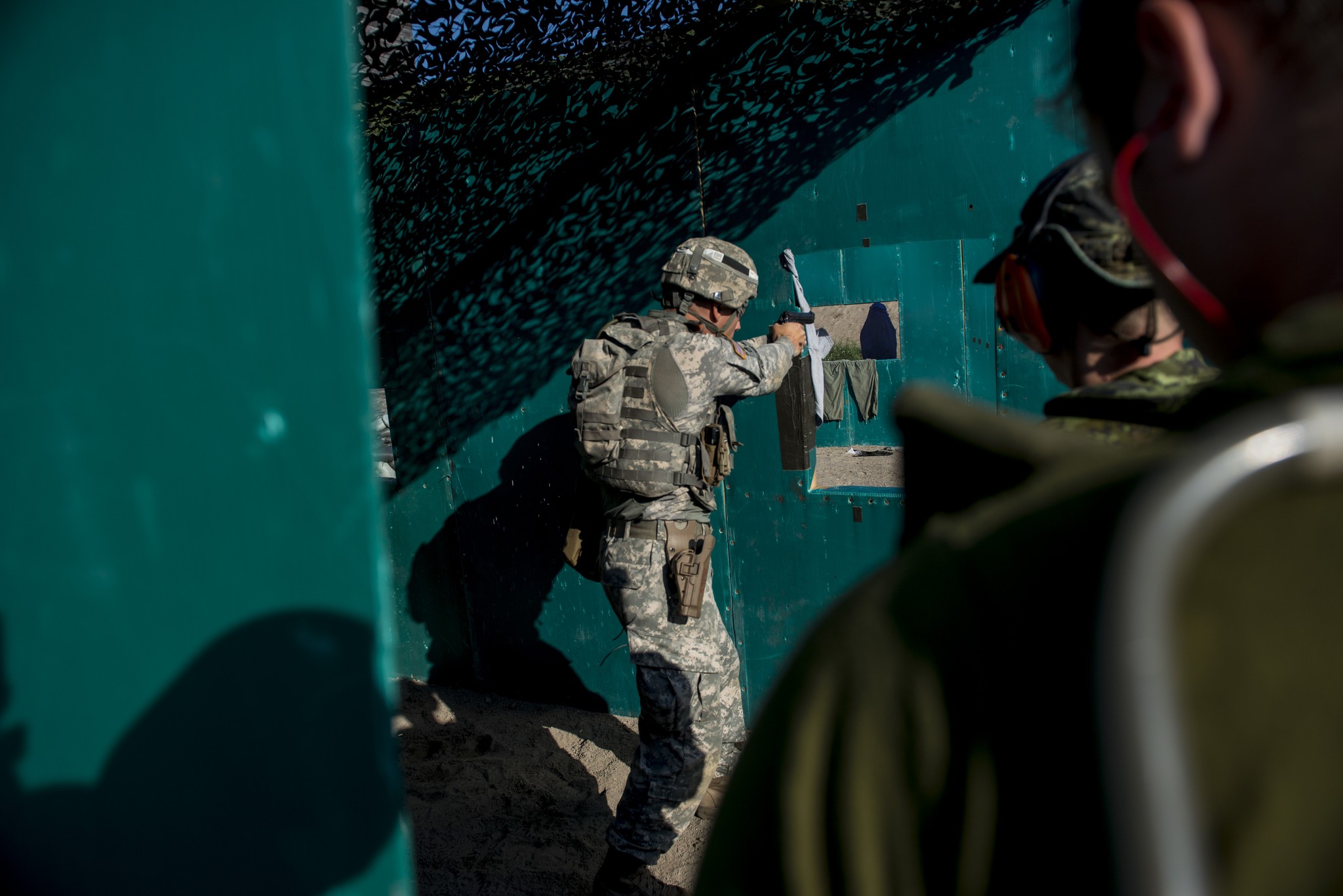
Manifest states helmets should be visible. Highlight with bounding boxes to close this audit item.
[660,238,759,309]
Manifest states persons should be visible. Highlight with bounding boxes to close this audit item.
[568,235,806,896]
[690,0,1343,896]
[971,150,1216,414]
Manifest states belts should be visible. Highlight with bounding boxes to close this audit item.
[608,519,659,540]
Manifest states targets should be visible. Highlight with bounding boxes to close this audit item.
[860,302,897,360]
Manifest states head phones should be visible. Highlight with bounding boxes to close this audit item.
[991,150,1090,361]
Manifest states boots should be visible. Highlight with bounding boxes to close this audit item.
[696,777,730,820]
[592,848,687,896]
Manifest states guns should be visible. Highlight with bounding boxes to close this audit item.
[778,309,815,325]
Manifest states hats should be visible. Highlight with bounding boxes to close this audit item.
[972,149,1157,290]
